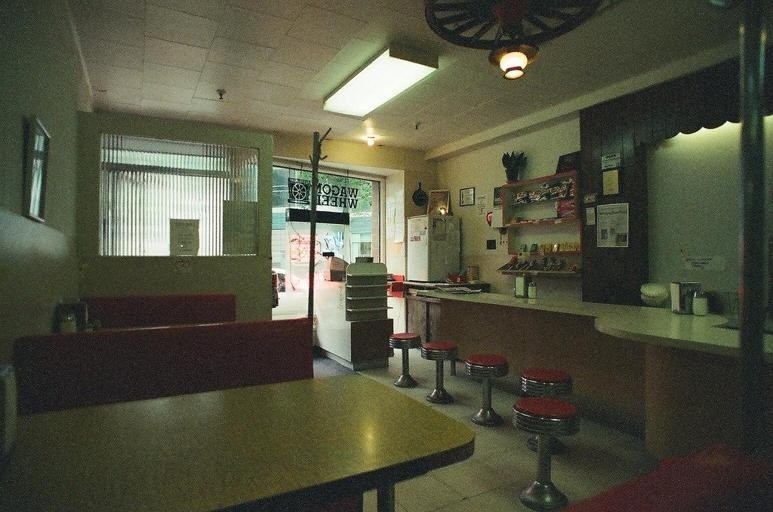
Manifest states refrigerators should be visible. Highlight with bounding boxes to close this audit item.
[406,213,462,282]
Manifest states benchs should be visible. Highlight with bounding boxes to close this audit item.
[11,294,315,464]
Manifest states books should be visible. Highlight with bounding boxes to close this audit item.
[434,286,474,295]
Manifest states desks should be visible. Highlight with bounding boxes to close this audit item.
[0,371,479,512]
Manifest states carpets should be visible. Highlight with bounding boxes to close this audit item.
[524,441,773,512]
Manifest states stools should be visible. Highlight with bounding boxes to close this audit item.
[389,329,580,511]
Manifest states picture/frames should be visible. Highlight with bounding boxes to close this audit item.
[425,189,451,215]
[23,113,54,224]
[555,151,584,172]
[458,187,476,207]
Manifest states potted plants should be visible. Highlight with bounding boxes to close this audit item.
[500,147,528,183]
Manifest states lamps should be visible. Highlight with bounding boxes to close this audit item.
[489,1,545,81]
[323,50,440,124]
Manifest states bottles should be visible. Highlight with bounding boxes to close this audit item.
[514,266,537,299]
[690,290,710,316]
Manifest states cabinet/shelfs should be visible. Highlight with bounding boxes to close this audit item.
[312,256,394,371]
[494,169,586,282]
